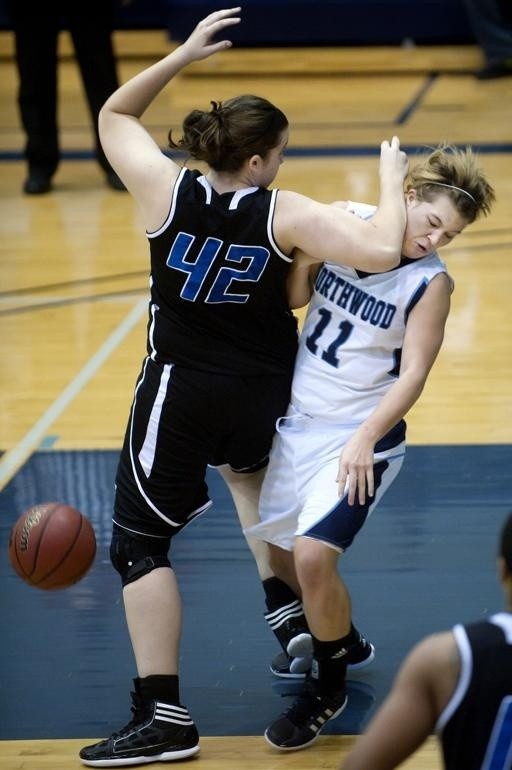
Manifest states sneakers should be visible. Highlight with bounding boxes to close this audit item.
[264,678,351,751]
[79,700,202,766]
[24,170,54,196]
[264,594,378,679]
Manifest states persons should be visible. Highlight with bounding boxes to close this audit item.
[332,507,512,770]
[239,136,499,758]
[76,3,411,767]
[10,1,138,198]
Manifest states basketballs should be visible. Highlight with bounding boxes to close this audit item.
[9,502,96,590]
